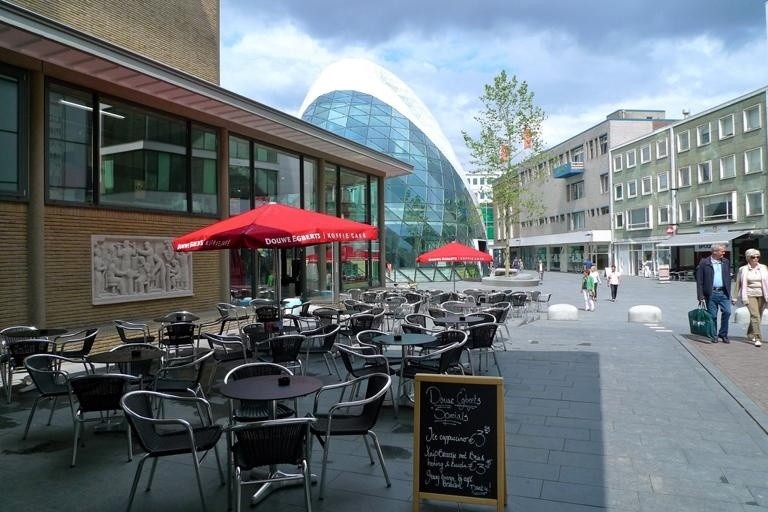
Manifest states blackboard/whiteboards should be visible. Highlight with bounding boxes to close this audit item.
[413,373,504,506]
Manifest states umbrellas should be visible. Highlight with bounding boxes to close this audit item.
[415,239,495,293]
[169,198,380,321]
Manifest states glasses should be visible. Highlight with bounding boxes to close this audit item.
[748,255,761,260]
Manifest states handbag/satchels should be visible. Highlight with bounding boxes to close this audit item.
[688,298,718,338]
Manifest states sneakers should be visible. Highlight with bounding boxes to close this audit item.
[753,339,762,347]
[584,308,595,312]
[592,297,598,302]
[745,335,756,342]
[712,335,718,343]
[610,298,615,303]
[721,335,730,344]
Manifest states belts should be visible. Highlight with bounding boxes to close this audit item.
[712,287,725,292]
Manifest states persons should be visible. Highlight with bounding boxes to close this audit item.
[580,269,595,312]
[732,248,768,348]
[607,264,621,301]
[537,260,545,281]
[694,240,733,344]
[589,265,602,299]
[511,256,520,269]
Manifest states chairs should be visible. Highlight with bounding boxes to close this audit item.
[670,269,690,283]
[120,389,223,508]
[1,286,511,477]
[223,413,319,509]
[508,288,550,325]
[302,372,390,500]
